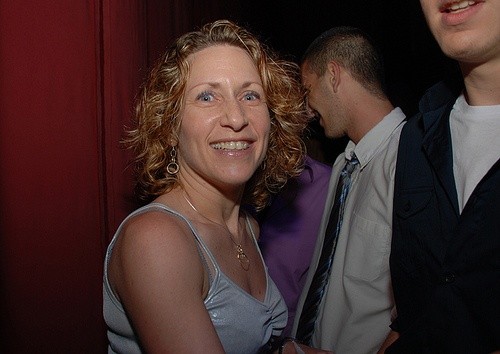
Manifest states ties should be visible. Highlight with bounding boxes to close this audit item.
[296,152,360,347]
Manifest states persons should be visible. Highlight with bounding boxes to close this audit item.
[384,0,500,354]
[104,20,335,354]
[290,28,407,354]
[246,154,332,339]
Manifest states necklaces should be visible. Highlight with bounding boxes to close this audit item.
[182,192,250,272]
